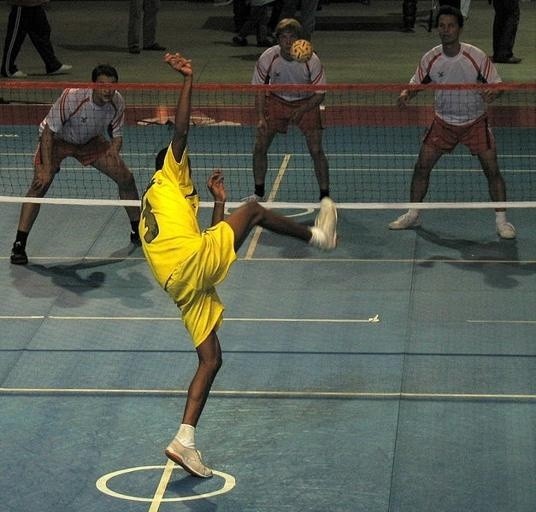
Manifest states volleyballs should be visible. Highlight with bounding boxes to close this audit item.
[290,39,312,62]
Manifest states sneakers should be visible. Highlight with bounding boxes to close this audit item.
[388,214,423,229]
[241,195,267,202]
[129,46,140,54]
[496,222,516,239]
[10,241,27,265]
[12,71,27,77]
[315,198,337,249]
[165,438,212,478]
[48,64,72,74]
[143,42,166,50]
[493,57,521,63]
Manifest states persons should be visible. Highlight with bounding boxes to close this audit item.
[241,18,330,202]
[268,1,317,40]
[388,7,516,238]
[232,1,276,47]
[0,1,72,79]
[136,53,337,479]
[10,64,142,263]
[128,0,168,54]
[492,0,522,63]
